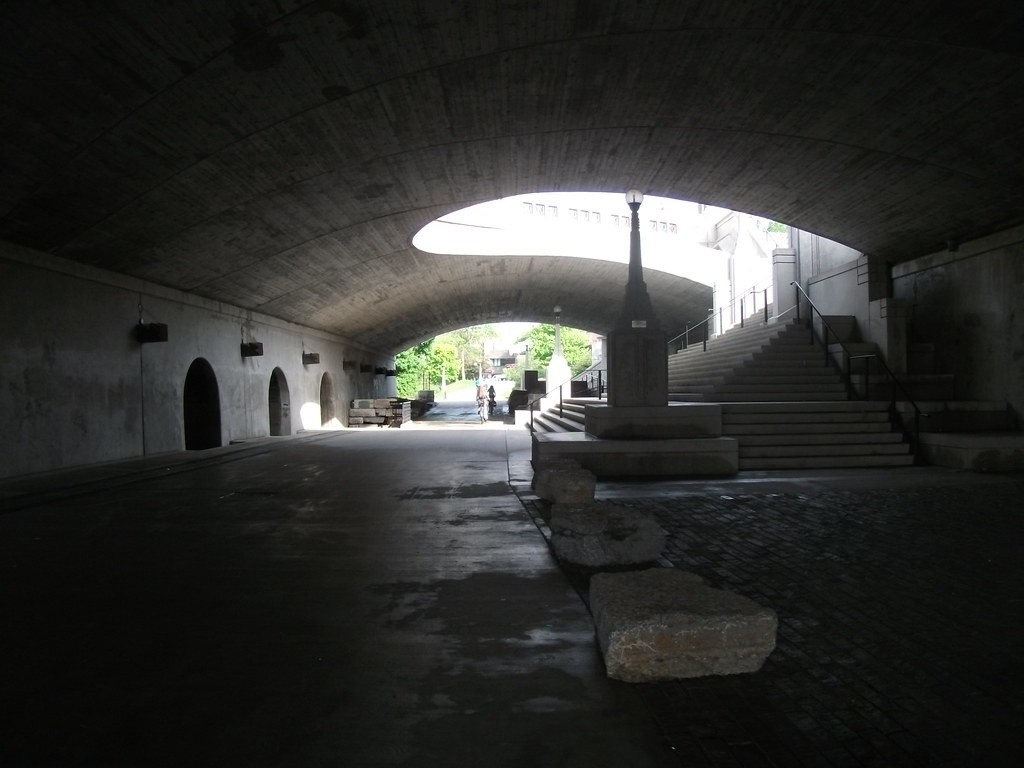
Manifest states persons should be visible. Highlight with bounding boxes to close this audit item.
[487,385,496,405]
[476,385,489,421]
